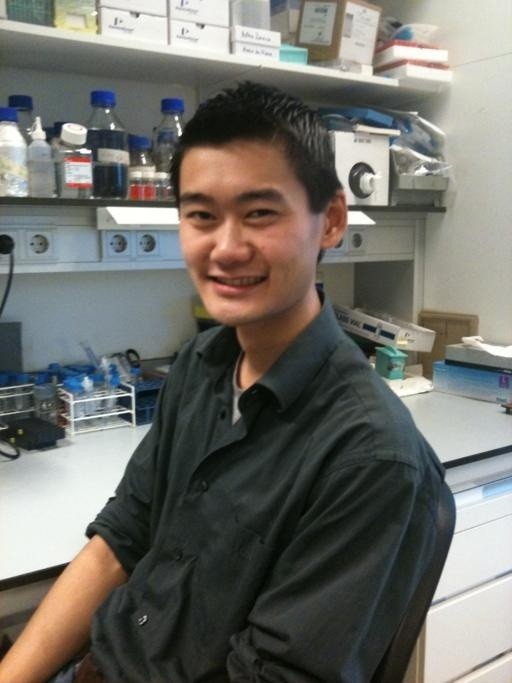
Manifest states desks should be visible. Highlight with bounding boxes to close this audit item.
[0,378,511,683]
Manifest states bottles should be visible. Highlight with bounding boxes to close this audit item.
[0,90,185,202]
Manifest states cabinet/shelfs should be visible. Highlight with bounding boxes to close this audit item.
[2,16,451,215]
[404,490,512,683]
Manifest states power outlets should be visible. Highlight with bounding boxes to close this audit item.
[330,227,366,258]
[99,231,166,263]
[0,217,61,264]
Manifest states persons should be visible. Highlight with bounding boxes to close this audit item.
[1,77,457,682]
